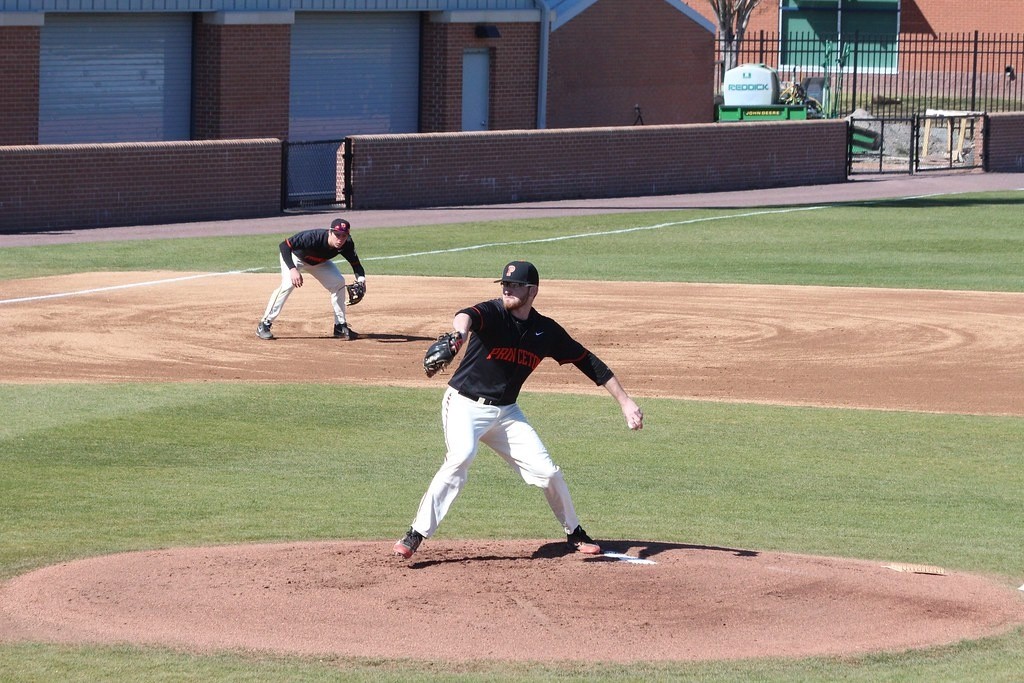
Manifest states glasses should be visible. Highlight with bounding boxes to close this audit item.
[501,281,537,289]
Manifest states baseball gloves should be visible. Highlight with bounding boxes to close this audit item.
[337,280,366,307]
[422,330,463,378]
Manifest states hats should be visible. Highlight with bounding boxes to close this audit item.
[494,260,539,284]
[330,219,350,235]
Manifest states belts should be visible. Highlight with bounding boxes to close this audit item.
[458,390,516,405]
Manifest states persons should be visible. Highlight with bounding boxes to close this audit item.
[392,259,644,559]
[255,217,367,341]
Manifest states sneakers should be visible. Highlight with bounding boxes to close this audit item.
[567,525,601,554]
[256,320,273,340]
[334,322,361,339]
[394,526,424,559]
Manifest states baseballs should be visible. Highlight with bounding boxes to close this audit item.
[627,419,638,429]
